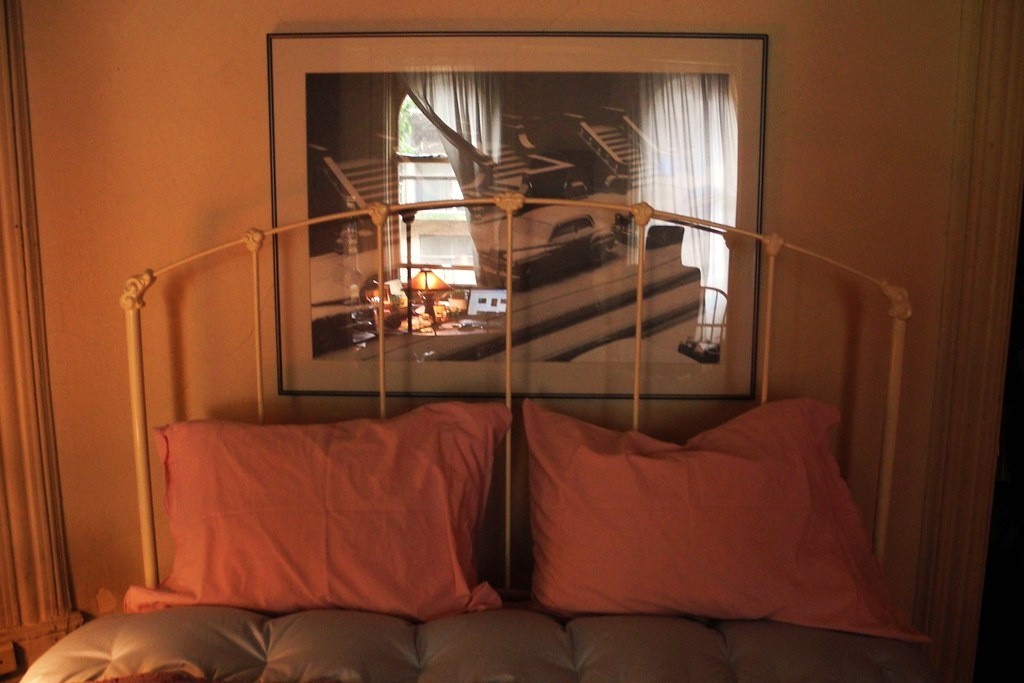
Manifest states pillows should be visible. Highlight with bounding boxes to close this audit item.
[121,402,514,623]
[520,396,933,644]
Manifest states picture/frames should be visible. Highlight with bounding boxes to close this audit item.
[265,32,769,402]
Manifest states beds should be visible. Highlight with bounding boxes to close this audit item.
[19,193,933,683]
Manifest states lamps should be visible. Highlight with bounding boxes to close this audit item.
[401,267,455,327]
